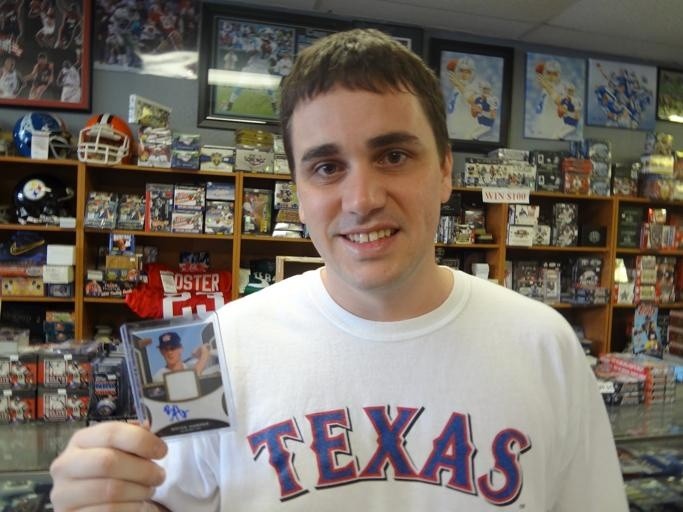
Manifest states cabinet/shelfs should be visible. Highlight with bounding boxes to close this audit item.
[237,169,312,306]
[432,182,504,288]
[482,191,616,355]
[0,153,81,348]
[578,193,682,356]
[75,157,239,344]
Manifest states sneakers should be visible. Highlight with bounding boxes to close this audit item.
[272,102,279,116]
[222,101,233,112]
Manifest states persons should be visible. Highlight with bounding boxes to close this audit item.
[47,25,632,511]
[218,21,296,88]
[594,63,653,129]
[6,358,83,422]
[220,43,279,115]
[152,330,212,388]
[94,1,199,69]
[1,0,83,102]
[529,58,582,140]
[446,57,499,140]
[633,315,659,356]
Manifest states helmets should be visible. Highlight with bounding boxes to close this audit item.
[12,113,71,160]
[542,60,561,86]
[13,173,76,225]
[455,56,476,85]
[77,114,133,166]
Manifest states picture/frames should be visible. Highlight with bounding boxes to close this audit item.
[0,0,95,115]
[189,3,424,139]
[427,35,515,156]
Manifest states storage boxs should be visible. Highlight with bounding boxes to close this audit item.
[132,122,292,177]
[0,240,73,299]
[504,202,577,252]
[458,134,681,200]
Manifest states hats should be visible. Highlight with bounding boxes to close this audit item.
[156,332,181,348]
